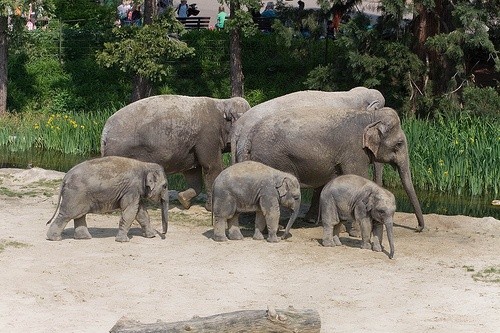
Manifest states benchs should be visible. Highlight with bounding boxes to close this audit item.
[176,17,210,30]
[225,16,287,30]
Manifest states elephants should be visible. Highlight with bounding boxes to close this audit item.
[228,86,389,221]
[210,160,302,244]
[43,154,171,243]
[242,105,426,233]
[94,94,253,215]
[313,172,398,260]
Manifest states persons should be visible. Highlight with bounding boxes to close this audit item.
[252,2,278,29]
[14,5,22,16]
[117,0,142,29]
[173,0,191,23]
[215,6,229,31]
